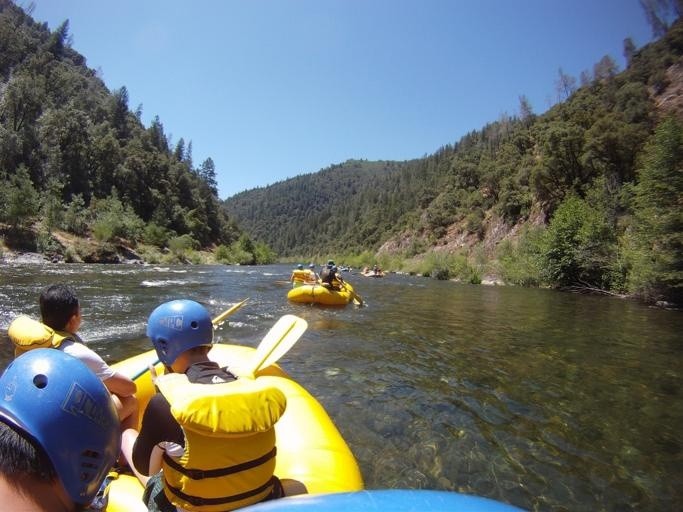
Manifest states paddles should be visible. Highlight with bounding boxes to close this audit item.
[131,297,249,378]
[247,315,308,378]
[275,281,315,285]
[340,281,363,306]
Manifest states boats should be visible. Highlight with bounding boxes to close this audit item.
[337,267,352,271]
[105,343,364,511]
[287,280,353,306]
[360,270,383,277]
[308,265,314,268]
[233,488,531,512]
[297,264,303,269]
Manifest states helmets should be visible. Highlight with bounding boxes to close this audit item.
[297,264,302,268]
[325,260,337,273]
[309,263,315,269]
[145,299,215,366]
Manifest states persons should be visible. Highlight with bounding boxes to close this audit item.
[290,264,306,287]
[318,259,342,292]
[303,264,315,285]
[13,283,138,478]
[120,299,286,511]
